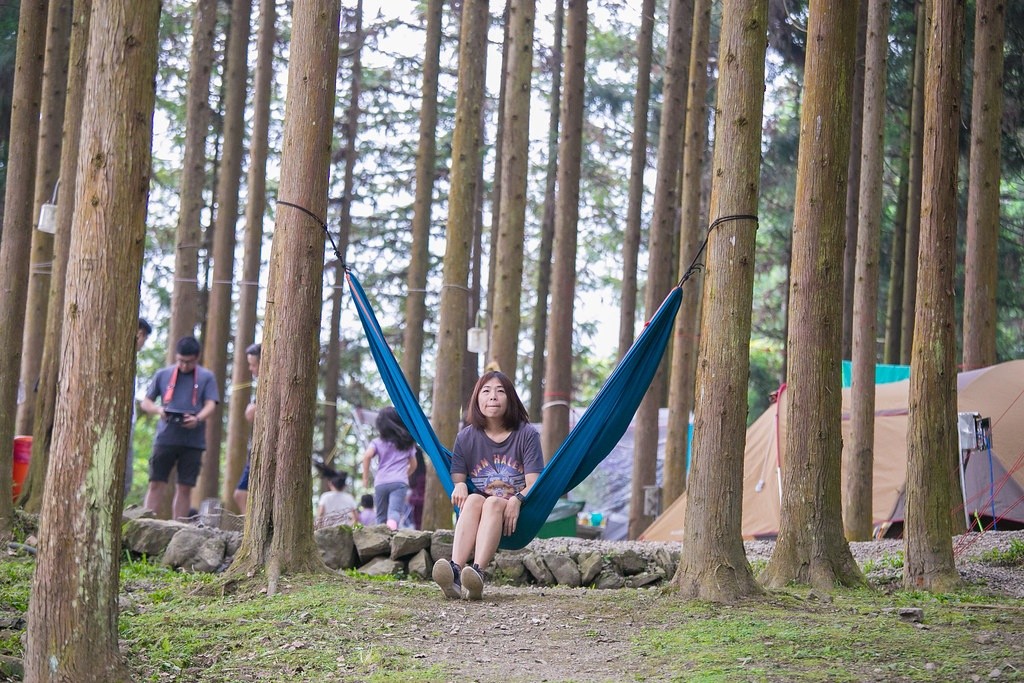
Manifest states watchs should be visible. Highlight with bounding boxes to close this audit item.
[515,492,526,502]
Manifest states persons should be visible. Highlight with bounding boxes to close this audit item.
[360,407,417,530]
[140,336,220,521]
[429,370,544,600]
[406,444,427,534]
[356,495,380,527]
[125,317,152,507]
[233,341,264,523]
[316,472,360,536]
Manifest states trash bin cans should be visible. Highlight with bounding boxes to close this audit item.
[13,434,33,503]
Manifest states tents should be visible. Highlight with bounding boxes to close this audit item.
[568,342,1024,554]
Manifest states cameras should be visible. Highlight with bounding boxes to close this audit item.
[164,407,186,425]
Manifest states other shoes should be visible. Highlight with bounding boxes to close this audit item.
[432,559,463,600]
[460,563,485,600]
